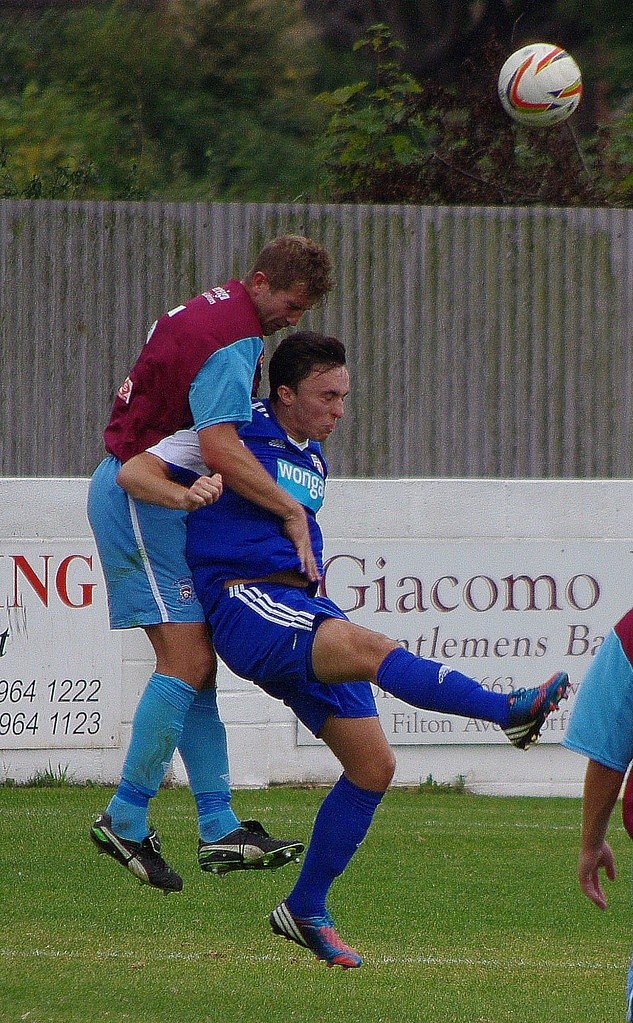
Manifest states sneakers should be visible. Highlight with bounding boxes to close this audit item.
[89,811,183,898]
[269,899,361,970]
[198,818,305,880]
[499,670,572,750]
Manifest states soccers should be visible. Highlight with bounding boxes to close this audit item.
[496,42,583,128]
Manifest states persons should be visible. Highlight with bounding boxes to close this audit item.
[563,610,633,1021]
[115,332,571,970]
[87,234,333,896]
